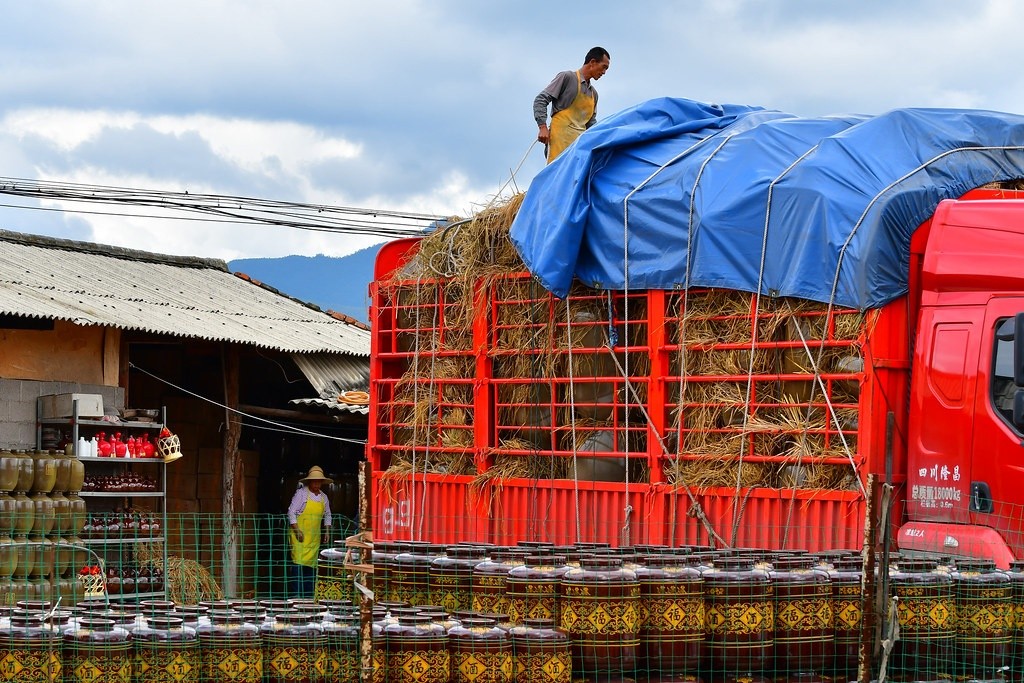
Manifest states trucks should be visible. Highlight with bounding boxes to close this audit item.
[363,186,1024,570]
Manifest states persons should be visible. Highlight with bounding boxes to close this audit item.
[533,46,611,167]
[287,466,333,598]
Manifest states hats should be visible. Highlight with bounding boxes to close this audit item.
[299,466,333,487]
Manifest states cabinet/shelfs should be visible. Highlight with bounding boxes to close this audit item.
[36,397,170,603]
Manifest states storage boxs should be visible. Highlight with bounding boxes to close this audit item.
[166,395,260,596]
[37,392,105,419]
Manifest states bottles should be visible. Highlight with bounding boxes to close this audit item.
[0,448,87,612]
[64,427,163,459]
